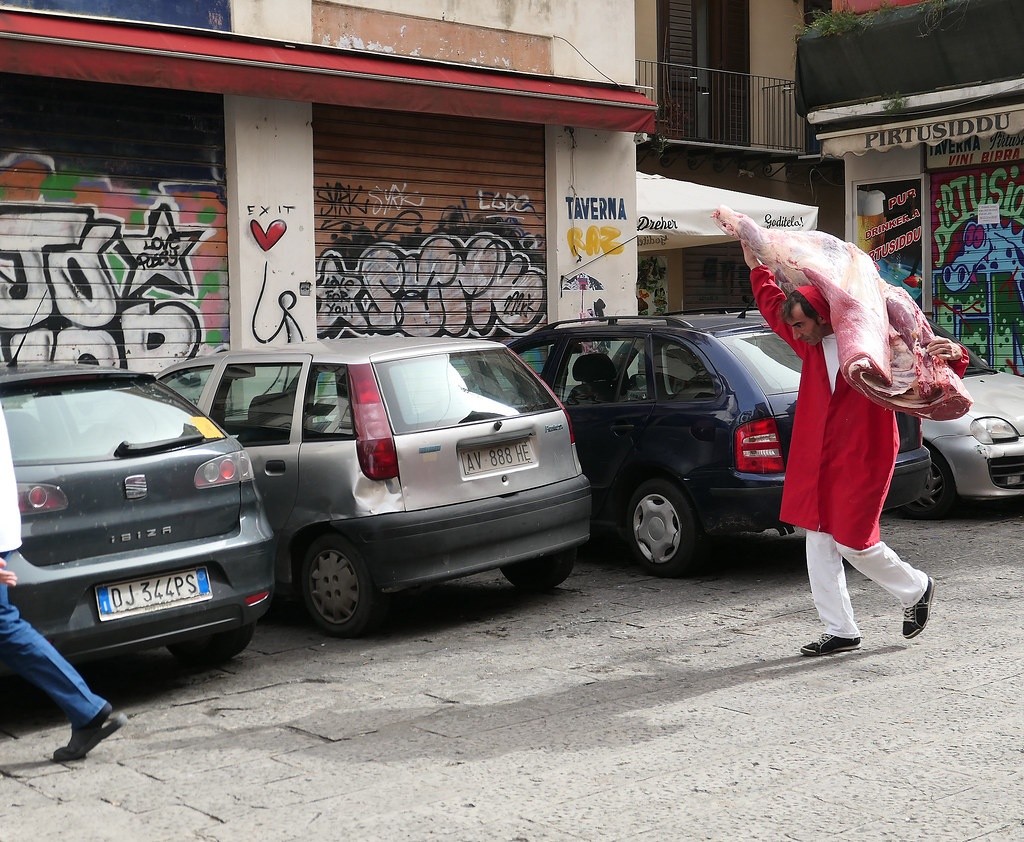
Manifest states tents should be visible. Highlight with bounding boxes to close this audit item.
[636,172,817,254]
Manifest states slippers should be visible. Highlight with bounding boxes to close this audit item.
[53,702,127,761]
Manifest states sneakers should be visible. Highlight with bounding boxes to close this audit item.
[903,576,935,639]
[800,633,861,656]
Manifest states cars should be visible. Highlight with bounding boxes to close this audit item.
[724,309,1024,521]
[155,336,593,639]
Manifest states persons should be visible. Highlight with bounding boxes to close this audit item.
[740,241,969,653]
[0,403,127,761]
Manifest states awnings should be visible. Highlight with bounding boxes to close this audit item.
[1,10,657,135]
[816,101,1024,160]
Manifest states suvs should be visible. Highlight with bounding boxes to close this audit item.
[1,361,278,679]
[463,305,932,576]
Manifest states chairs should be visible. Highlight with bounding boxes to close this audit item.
[562,353,622,406]
[245,390,296,442]
